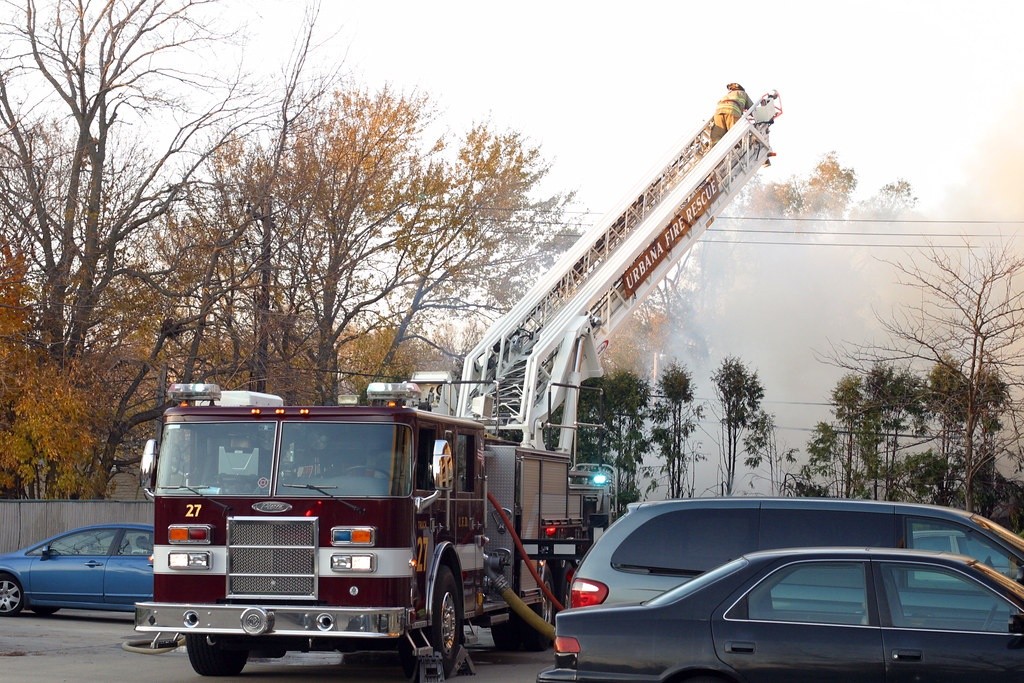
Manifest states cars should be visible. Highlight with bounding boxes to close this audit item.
[535,546,1024,683]
[0,522,154,616]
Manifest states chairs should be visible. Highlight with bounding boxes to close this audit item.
[121,536,149,556]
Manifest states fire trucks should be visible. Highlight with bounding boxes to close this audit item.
[134,90,783,678]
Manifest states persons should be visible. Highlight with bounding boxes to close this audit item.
[711,83,757,147]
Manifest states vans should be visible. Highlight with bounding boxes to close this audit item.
[567,498,1024,618]
